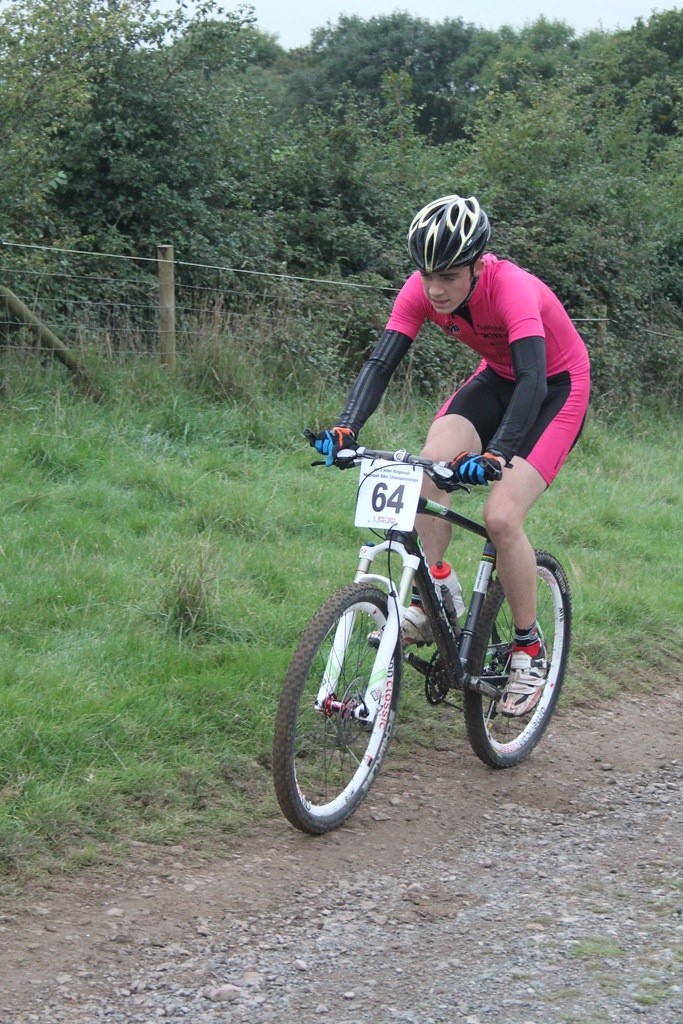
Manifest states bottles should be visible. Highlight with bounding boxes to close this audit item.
[430,560,465,619]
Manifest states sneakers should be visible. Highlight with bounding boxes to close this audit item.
[499,645,548,716]
[367,607,434,650]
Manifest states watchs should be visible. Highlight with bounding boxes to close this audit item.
[489,449,513,469]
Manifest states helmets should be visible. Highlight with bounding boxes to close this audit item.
[407,194,492,274]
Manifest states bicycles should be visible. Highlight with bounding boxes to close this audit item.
[272,429,574,837]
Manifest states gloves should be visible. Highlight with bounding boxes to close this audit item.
[314,427,355,467]
[447,450,503,486]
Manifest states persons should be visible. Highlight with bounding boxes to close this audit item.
[310,192,590,718]
